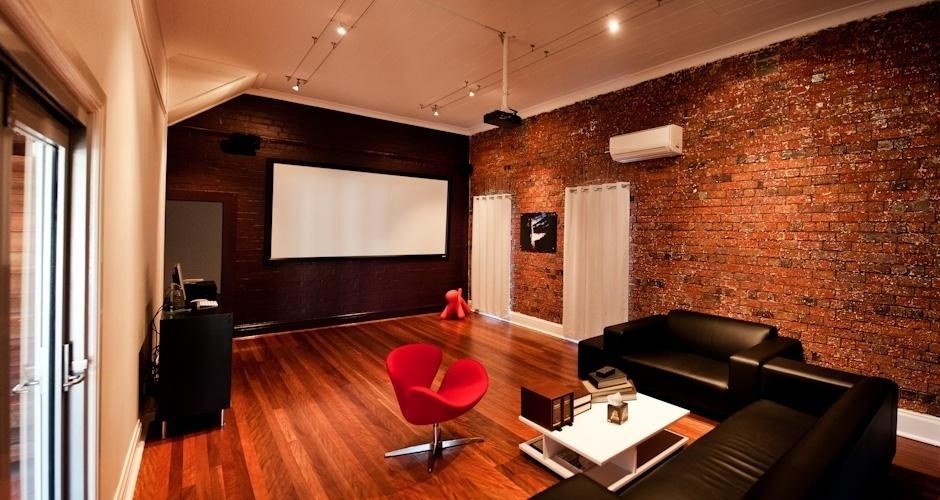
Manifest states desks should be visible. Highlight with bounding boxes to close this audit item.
[156,295,233,440]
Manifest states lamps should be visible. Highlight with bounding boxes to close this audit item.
[292,79,301,92]
[433,104,439,115]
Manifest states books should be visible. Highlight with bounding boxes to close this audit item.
[581,364,637,403]
[531,436,543,455]
[566,390,592,416]
[555,451,595,473]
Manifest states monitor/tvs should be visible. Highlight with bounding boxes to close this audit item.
[170,263,187,303]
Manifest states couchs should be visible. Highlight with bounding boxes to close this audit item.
[578,306,805,424]
[527,357,900,500]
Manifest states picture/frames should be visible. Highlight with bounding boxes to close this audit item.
[519,213,558,255]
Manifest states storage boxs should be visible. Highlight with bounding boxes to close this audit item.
[521,380,574,431]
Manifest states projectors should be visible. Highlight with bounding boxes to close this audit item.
[483,108,523,129]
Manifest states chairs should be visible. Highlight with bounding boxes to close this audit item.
[384,342,489,473]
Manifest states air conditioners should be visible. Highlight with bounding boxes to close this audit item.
[609,124,682,163]
